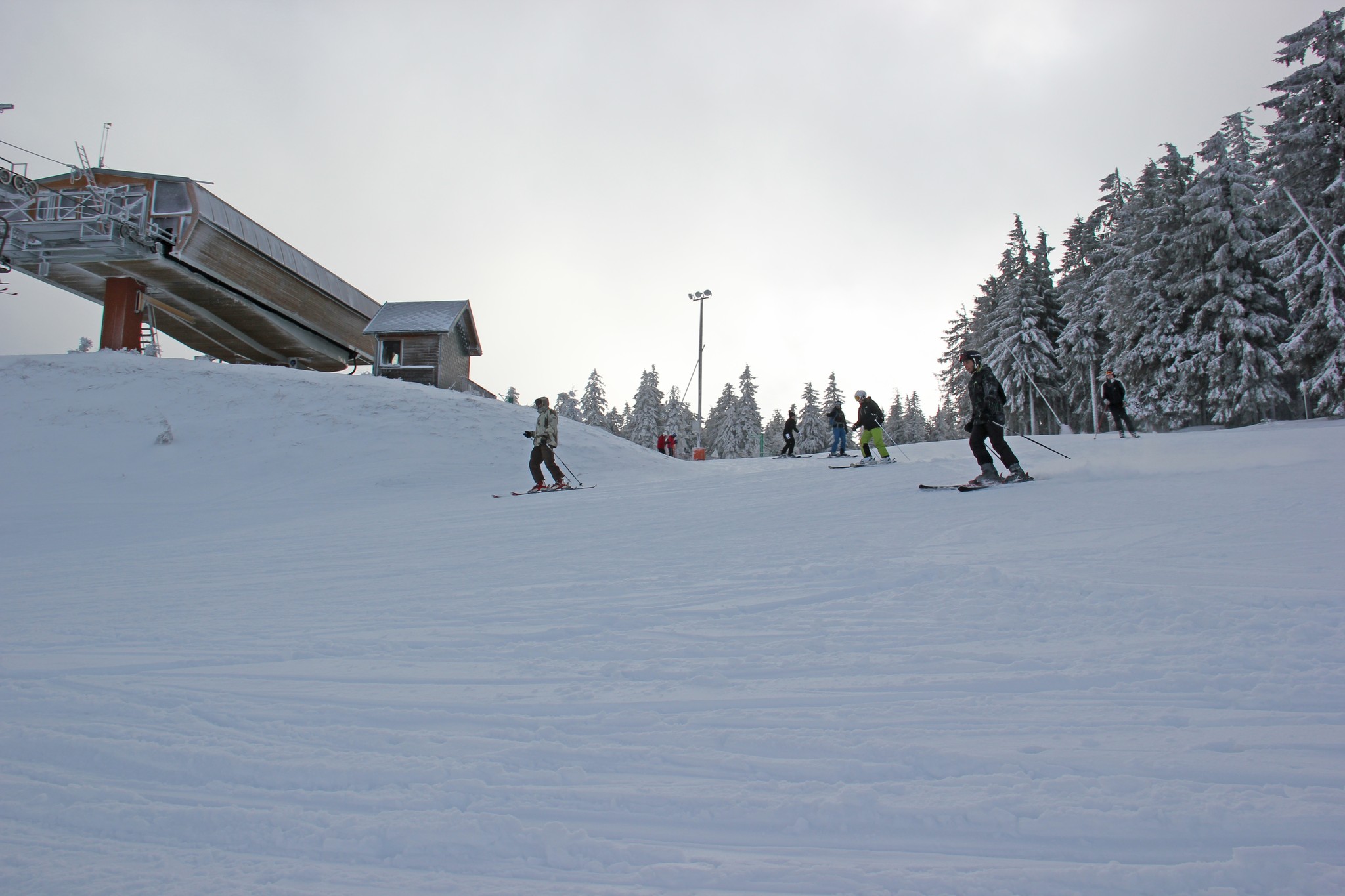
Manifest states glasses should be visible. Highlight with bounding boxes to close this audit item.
[855,396,861,401]
[535,400,541,405]
[959,354,967,362]
[1106,371,1113,375]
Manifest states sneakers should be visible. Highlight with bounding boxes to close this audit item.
[968,463,999,484]
[860,456,876,464]
[838,452,850,456]
[1005,462,1026,481]
[532,480,547,492]
[829,453,840,457]
[551,478,566,490]
[877,456,892,465]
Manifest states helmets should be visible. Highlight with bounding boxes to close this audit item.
[963,349,981,363]
[855,390,866,399]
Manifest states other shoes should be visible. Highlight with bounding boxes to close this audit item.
[780,454,786,458]
[1133,435,1140,438]
[788,453,795,457]
[1120,434,1124,438]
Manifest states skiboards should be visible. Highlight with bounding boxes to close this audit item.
[1119,435,1141,438]
[817,455,858,458]
[772,455,813,459]
[919,478,1052,492]
[0,288,18,295]
[828,461,897,469]
[491,484,597,498]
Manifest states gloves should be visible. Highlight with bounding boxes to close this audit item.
[523,430,531,438]
[844,415,878,434]
[798,431,803,436]
[1104,399,1110,405]
[979,407,989,421]
[786,433,791,440]
[964,422,972,433]
[540,435,547,444]
[1123,402,1127,407]
[675,440,678,444]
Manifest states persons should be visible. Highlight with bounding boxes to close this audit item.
[960,349,1028,484]
[154,228,174,258]
[780,412,802,457]
[524,397,568,493]
[826,401,849,457]
[658,432,670,454]
[1100,370,1138,438]
[852,390,891,464]
[668,433,678,457]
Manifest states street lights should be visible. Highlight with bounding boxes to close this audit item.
[687,289,712,463]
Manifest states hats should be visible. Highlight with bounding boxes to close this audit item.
[673,433,677,437]
[789,410,795,417]
[835,401,841,405]
